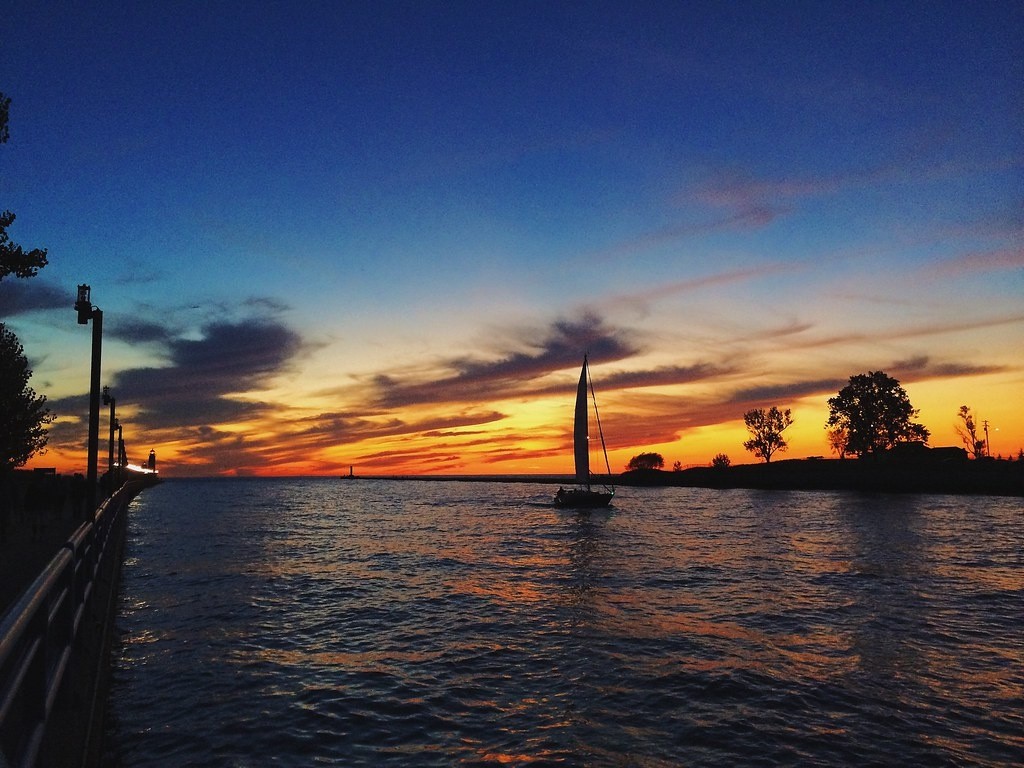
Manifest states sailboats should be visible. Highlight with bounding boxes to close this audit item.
[555,352,615,506]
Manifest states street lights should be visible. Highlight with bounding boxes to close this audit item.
[102,384,128,498]
[75,284,103,524]
